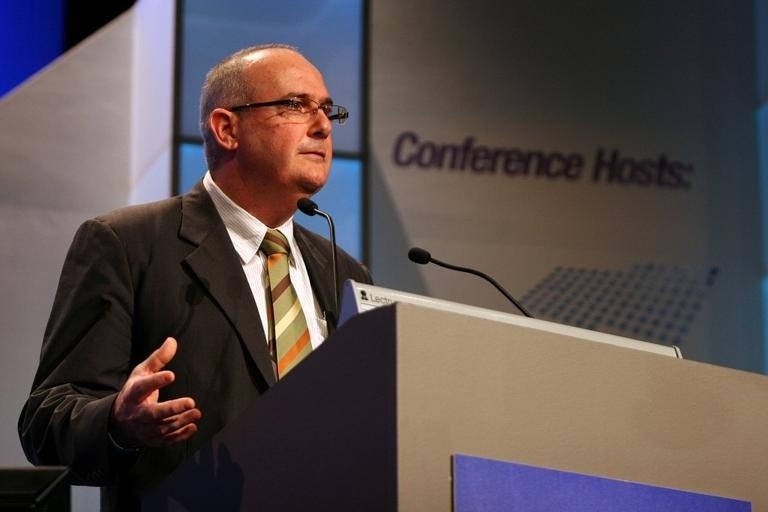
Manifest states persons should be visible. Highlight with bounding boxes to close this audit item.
[18,44,375,512]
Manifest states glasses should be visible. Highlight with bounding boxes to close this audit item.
[226,95,349,126]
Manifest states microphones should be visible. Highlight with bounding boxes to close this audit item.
[297,196,341,319]
[408,247,537,319]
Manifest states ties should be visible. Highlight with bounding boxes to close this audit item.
[256,228,314,380]
